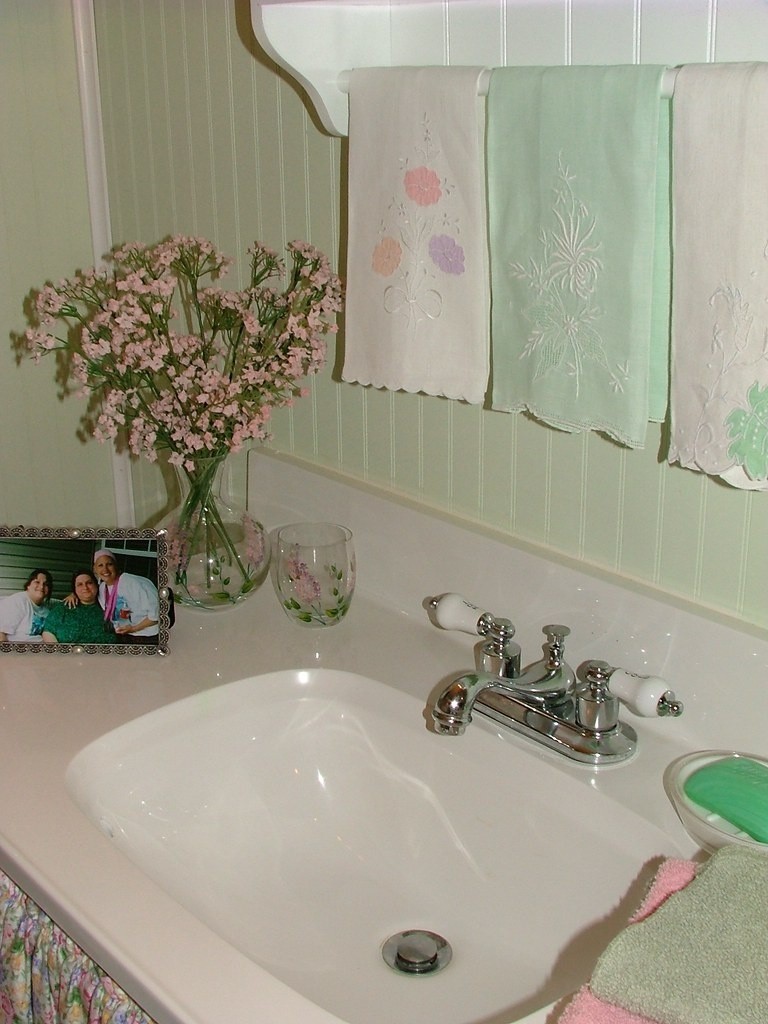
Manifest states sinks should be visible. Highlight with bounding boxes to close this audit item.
[61,670,690,1024]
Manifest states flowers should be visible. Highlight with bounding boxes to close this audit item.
[24,233,363,590]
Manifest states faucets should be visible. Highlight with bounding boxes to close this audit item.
[428,591,685,765]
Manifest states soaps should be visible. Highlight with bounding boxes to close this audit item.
[684,756,768,844]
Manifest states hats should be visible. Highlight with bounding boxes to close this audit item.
[94,549,114,564]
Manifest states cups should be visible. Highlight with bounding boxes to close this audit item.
[275,522,357,629]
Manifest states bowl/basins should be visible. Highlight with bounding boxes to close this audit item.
[668,750,768,856]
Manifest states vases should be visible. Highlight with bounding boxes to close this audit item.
[150,453,271,613]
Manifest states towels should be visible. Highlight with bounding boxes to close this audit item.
[339,63,492,406]
[665,61,768,494]
[588,843,768,1024]
[557,856,768,1024]
[483,64,673,452]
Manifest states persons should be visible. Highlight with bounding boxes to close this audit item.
[42,569,119,645]
[0,567,64,642]
[62,548,159,645]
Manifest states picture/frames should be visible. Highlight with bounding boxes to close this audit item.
[0,526,170,657]
